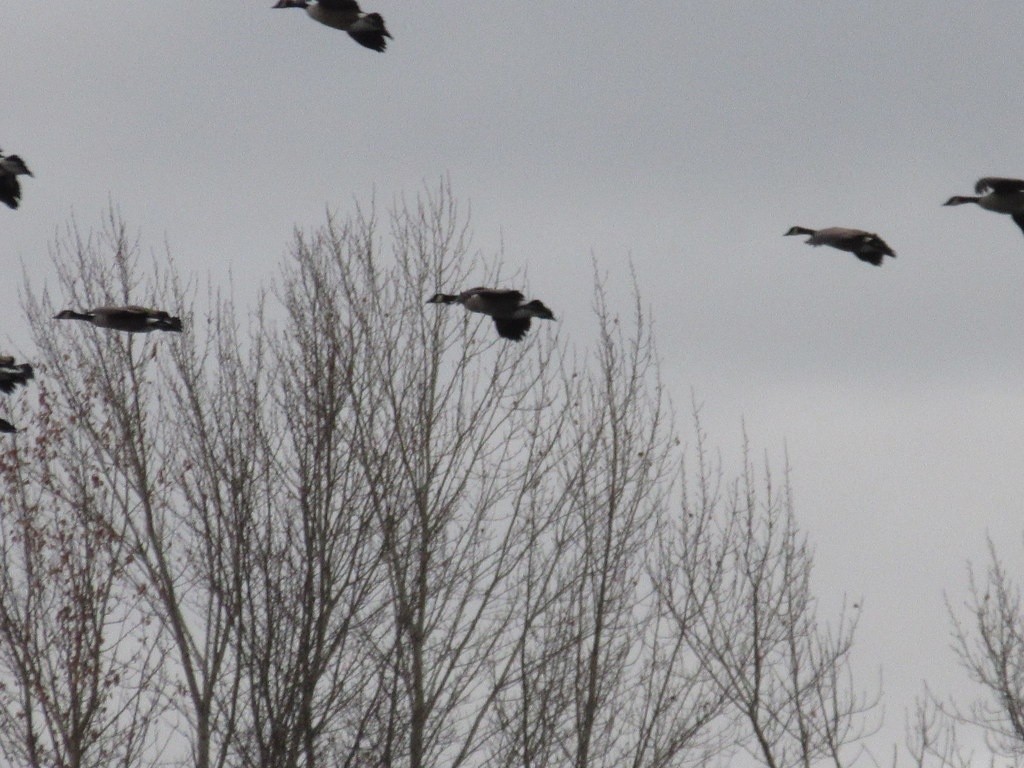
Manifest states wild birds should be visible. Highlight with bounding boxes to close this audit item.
[0,352,35,434]
[268,0,394,53]
[940,176,1024,235]
[425,286,558,343]
[51,303,183,333]
[0,149,34,211]
[782,225,898,267]
